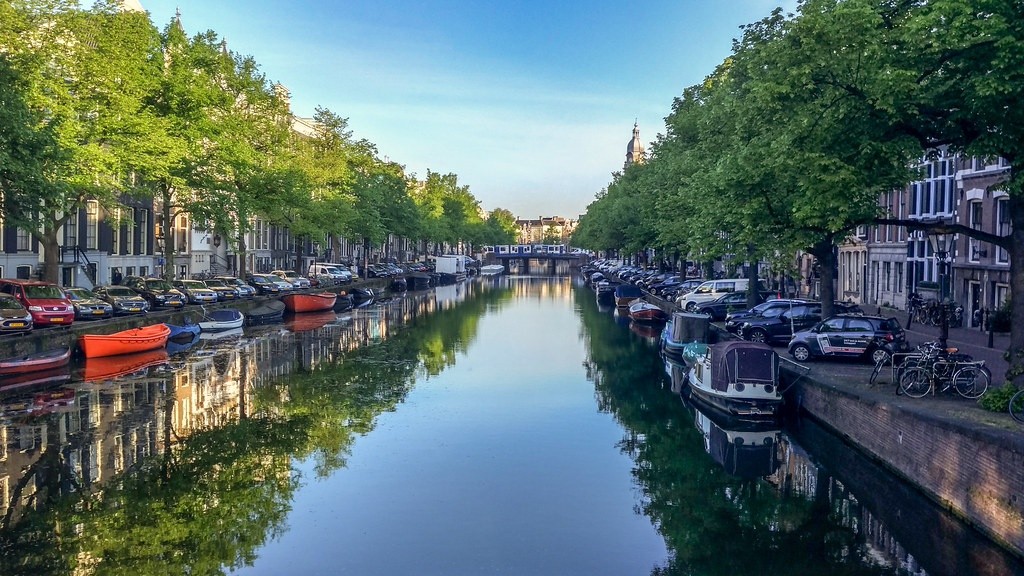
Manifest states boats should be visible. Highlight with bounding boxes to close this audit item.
[168,308,243,338]
[245,298,284,320]
[282,290,337,312]
[581,266,615,297]
[80,323,169,358]
[334,286,374,303]
[481,263,504,275]
[682,338,783,417]
[613,284,662,322]
[392,272,467,288]
[0,344,71,374]
[660,311,711,354]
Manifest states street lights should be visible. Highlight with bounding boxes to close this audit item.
[927,215,957,354]
[156,234,165,277]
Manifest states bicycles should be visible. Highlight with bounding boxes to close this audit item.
[906,296,946,327]
[313,274,335,289]
[869,339,1024,425]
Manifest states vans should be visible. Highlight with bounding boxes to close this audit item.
[441,255,475,266]
[0,277,74,328]
[308,263,353,284]
[571,248,589,257]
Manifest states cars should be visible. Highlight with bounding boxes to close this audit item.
[0,270,257,333]
[351,261,436,281]
[249,269,311,294]
[678,278,909,362]
[590,257,703,301]
